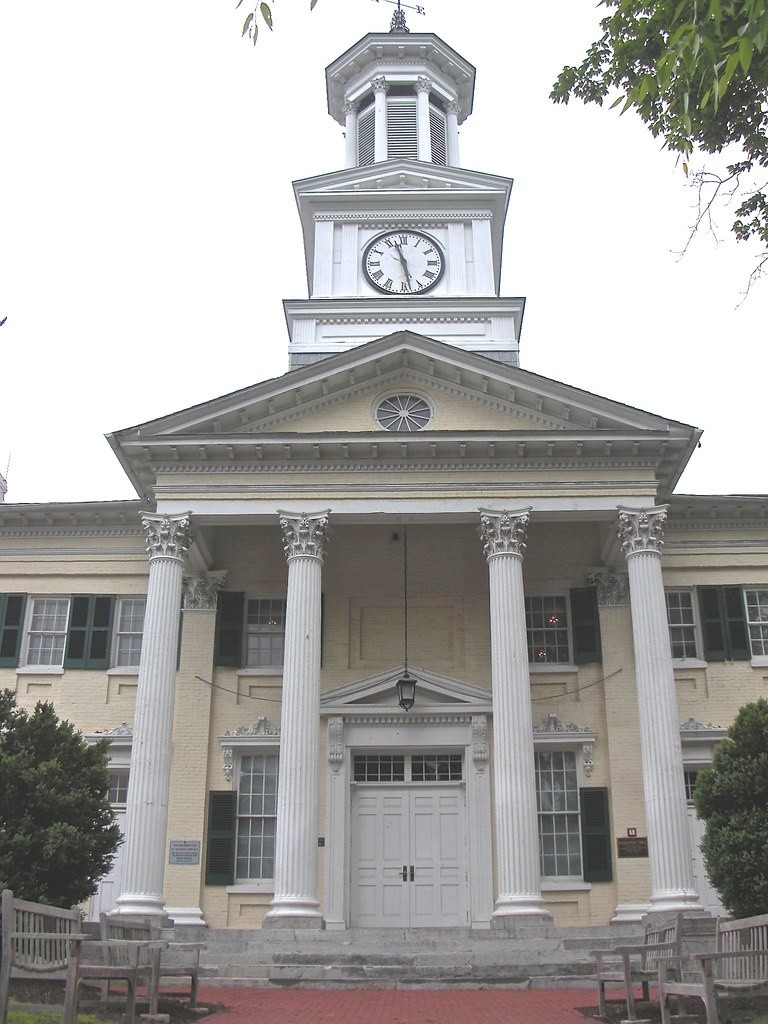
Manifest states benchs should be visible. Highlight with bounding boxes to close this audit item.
[0,890,149,1024]
[99,913,206,1014]
[588,913,685,1020]
[646,912,768,1024]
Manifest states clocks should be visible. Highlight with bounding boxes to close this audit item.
[361,231,446,294]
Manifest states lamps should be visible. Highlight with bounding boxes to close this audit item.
[395,525,417,712]
